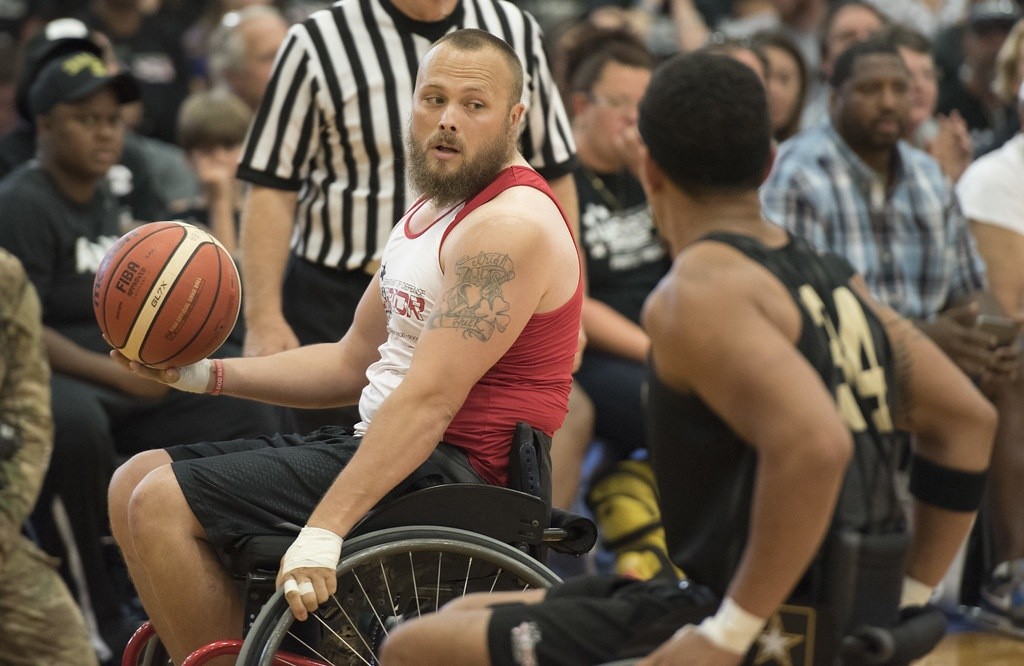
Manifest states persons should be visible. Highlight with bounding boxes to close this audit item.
[234,3,583,356]
[0,0,1022,666]
[105,38,585,664]
[377,54,1001,666]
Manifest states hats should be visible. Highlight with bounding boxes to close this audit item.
[22,54,148,119]
[14,37,106,117]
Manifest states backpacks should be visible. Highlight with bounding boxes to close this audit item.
[584,454,691,589]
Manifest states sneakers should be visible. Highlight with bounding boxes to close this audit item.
[973,554,1024,641]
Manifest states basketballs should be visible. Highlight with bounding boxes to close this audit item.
[89,217,244,372]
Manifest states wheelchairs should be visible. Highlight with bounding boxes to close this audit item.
[120,421,596,666]
